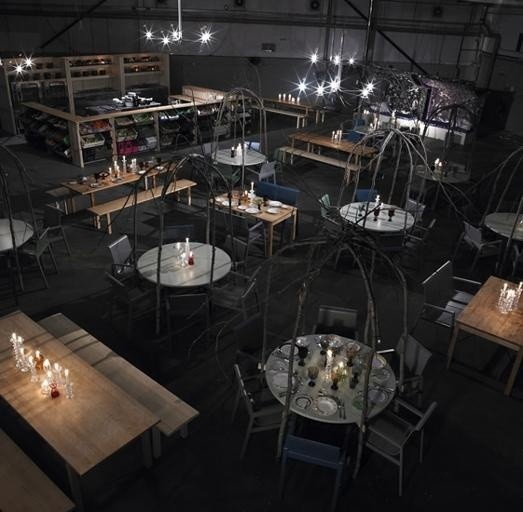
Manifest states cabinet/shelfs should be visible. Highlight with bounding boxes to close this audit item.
[0,54,251,168]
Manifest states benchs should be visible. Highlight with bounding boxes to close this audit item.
[35,311,202,458]
[0,428,75,511]
[244,102,309,132]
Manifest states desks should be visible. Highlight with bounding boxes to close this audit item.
[248,95,332,129]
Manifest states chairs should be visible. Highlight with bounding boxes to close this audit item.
[2,120,522,343]
[276,434,353,511]
[311,304,360,343]
[236,312,287,372]
[358,399,438,499]
[1,311,161,501]
[233,365,305,461]
[410,271,462,356]
[371,333,430,415]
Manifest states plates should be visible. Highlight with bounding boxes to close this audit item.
[215,193,288,215]
[268,334,391,417]
